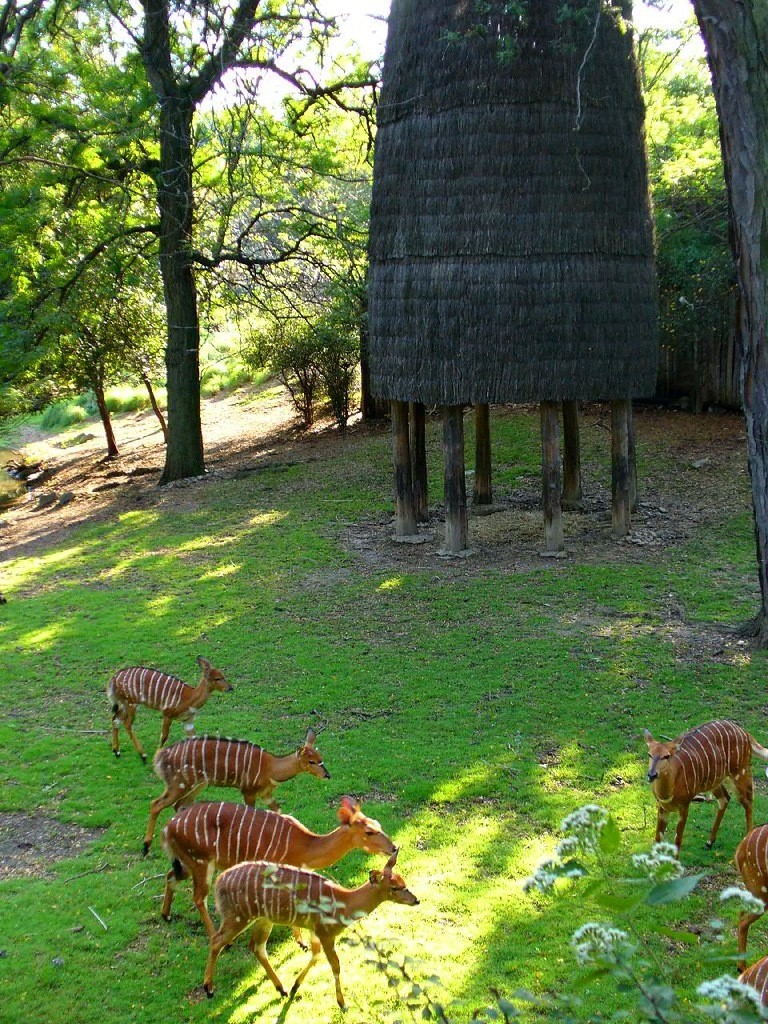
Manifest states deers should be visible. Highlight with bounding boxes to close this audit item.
[106,655,421,1010]
[644,719,768,1013]
